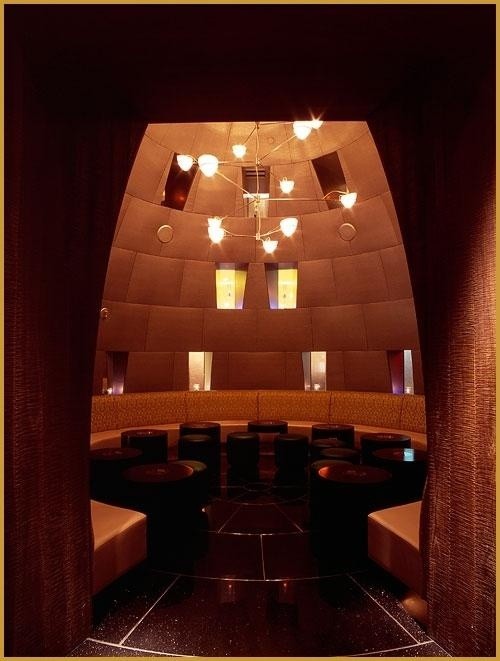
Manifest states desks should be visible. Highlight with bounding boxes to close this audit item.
[89,448,144,507]
[247,420,288,435]
[120,429,167,464]
[312,424,355,448]
[179,422,221,475]
[123,464,194,571]
[373,448,427,503]
[360,434,411,468]
[319,465,391,543]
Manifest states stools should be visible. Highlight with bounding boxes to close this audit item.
[227,432,259,480]
[311,439,344,461]
[178,434,214,482]
[167,459,209,528]
[320,448,361,464]
[309,460,351,472]
[272,433,309,480]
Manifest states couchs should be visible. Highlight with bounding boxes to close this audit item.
[90,390,429,623]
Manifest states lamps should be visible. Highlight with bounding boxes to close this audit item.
[177,122,358,253]
